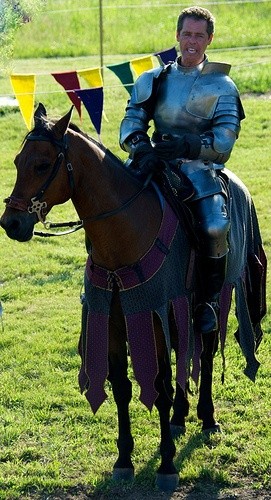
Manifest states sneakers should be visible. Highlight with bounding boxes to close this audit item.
[195,296,219,334]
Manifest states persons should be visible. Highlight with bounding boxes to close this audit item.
[119,9,245,332]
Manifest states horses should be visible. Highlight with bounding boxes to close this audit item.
[0,102,267,491]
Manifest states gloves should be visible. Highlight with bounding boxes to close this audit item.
[133,143,165,178]
[152,132,191,159]
